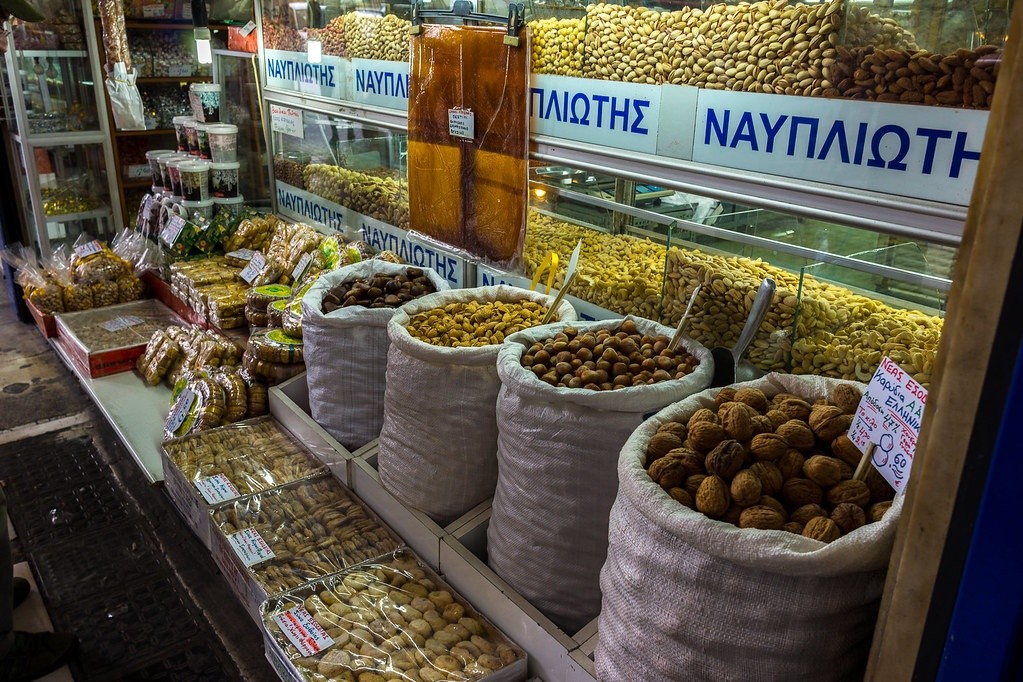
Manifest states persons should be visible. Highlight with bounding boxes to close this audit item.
[0,0,79,681]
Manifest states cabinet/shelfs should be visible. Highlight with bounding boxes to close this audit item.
[0,0,272,263]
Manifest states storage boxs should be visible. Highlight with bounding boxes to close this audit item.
[267,369,379,488]
[209,471,405,632]
[350,445,492,574]
[439,508,599,682]
[258,547,529,682]
[23,291,56,338]
[54,298,192,379]
[160,415,329,551]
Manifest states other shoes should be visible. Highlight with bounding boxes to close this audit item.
[12,577,30,608]
[0,631,78,682]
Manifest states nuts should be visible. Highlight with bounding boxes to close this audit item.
[27,249,144,317]
[258,0,1010,546]
